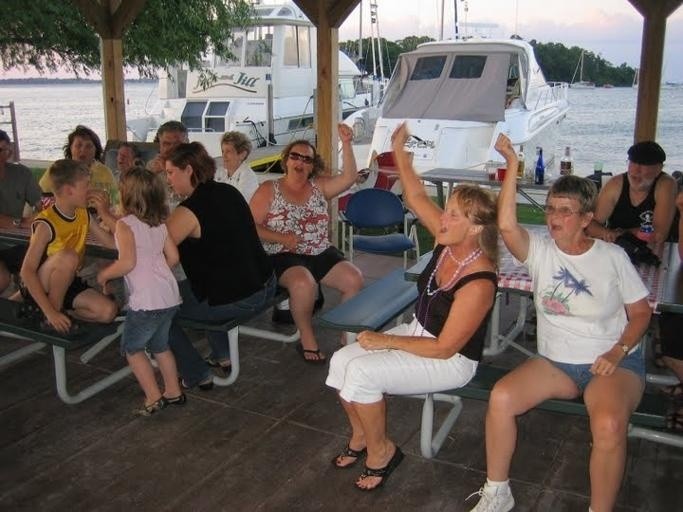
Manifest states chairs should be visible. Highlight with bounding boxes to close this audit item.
[336,151,420,270]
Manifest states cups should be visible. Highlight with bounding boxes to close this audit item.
[39,192,54,211]
[487,167,505,181]
[593,161,603,173]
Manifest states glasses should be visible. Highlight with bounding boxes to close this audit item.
[288,150,313,164]
[541,204,573,218]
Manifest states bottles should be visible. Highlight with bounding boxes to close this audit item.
[637,214,655,251]
[560,146,571,177]
[533,148,544,184]
[516,143,525,179]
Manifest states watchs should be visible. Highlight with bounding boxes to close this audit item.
[616,342,629,355]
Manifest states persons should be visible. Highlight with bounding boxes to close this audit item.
[586,140,678,259]
[652,172,683,430]
[465,134,654,511]
[334,119,500,491]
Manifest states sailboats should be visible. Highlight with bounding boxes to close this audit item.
[570,49,595,90]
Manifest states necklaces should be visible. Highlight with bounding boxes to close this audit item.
[411,247,482,337]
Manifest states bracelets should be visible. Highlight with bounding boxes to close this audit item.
[387,334,392,350]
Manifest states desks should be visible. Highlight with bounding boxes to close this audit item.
[419,168,556,213]
[17,159,55,169]
[1,211,118,258]
[212,144,285,184]
[405,221,681,385]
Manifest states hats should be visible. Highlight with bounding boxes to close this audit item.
[628,141,667,165]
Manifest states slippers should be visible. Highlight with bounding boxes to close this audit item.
[353,444,406,494]
[329,438,369,470]
[297,341,326,364]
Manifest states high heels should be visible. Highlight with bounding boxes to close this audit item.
[178,375,214,391]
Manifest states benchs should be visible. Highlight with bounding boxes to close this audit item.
[0,285,301,404]
[319,272,419,345]
[400,362,682,456]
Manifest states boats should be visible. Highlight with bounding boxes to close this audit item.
[604,84,614,89]
[342,1,572,188]
[123,1,389,181]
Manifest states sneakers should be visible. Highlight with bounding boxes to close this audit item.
[131,396,169,417]
[467,481,515,512]
[162,392,186,405]
